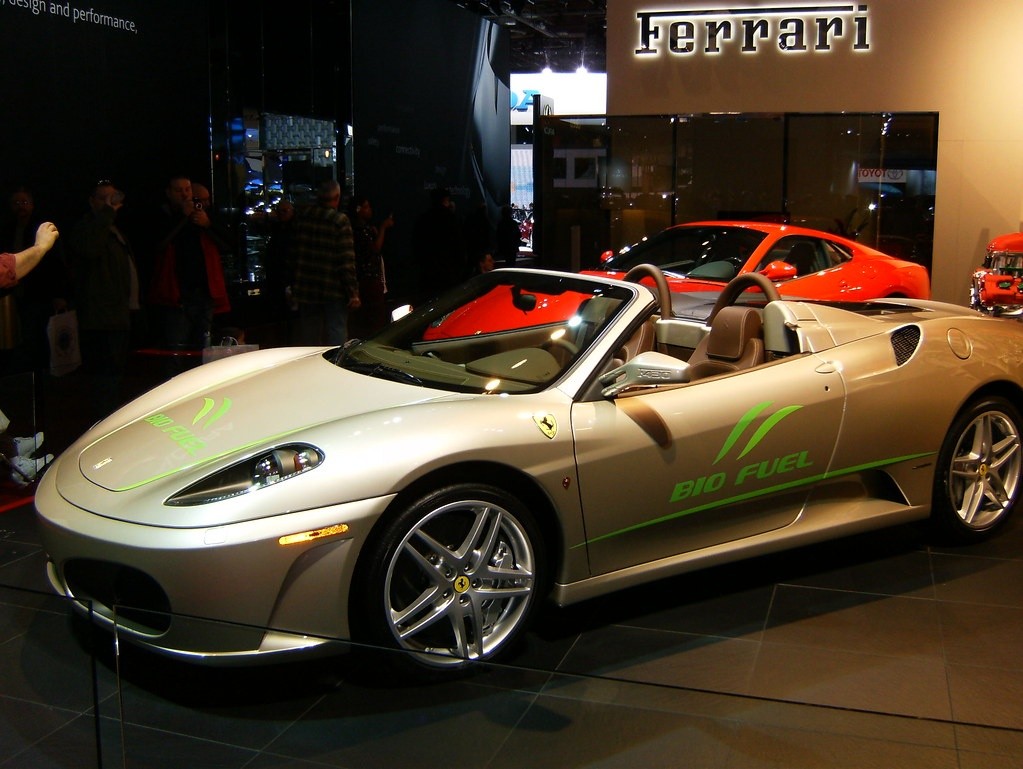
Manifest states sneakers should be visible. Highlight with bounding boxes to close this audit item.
[8,431,44,463]
[11,454,54,486]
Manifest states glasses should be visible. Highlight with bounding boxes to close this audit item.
[10,199,34,208]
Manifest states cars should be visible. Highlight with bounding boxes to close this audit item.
[970,234,1023,320]
[511,209,534,243]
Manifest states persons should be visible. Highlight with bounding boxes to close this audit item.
[475,250,495,275]
[253,179,394,347]
[0,182,61,489]
[74,170,143,411]
[493,204,522,268]
[160,173,229,364]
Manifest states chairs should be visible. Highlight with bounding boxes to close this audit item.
[785,240,820,275]
[687,306,763,380]
[595,320,658,373]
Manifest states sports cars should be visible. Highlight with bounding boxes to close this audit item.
[421,219,931,340]
[32,262,1023,685]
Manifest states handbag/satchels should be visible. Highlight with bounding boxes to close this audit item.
[202,336,260,365]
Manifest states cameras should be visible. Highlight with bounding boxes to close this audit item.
[110,191,124,206]
[192,196,203,211]
